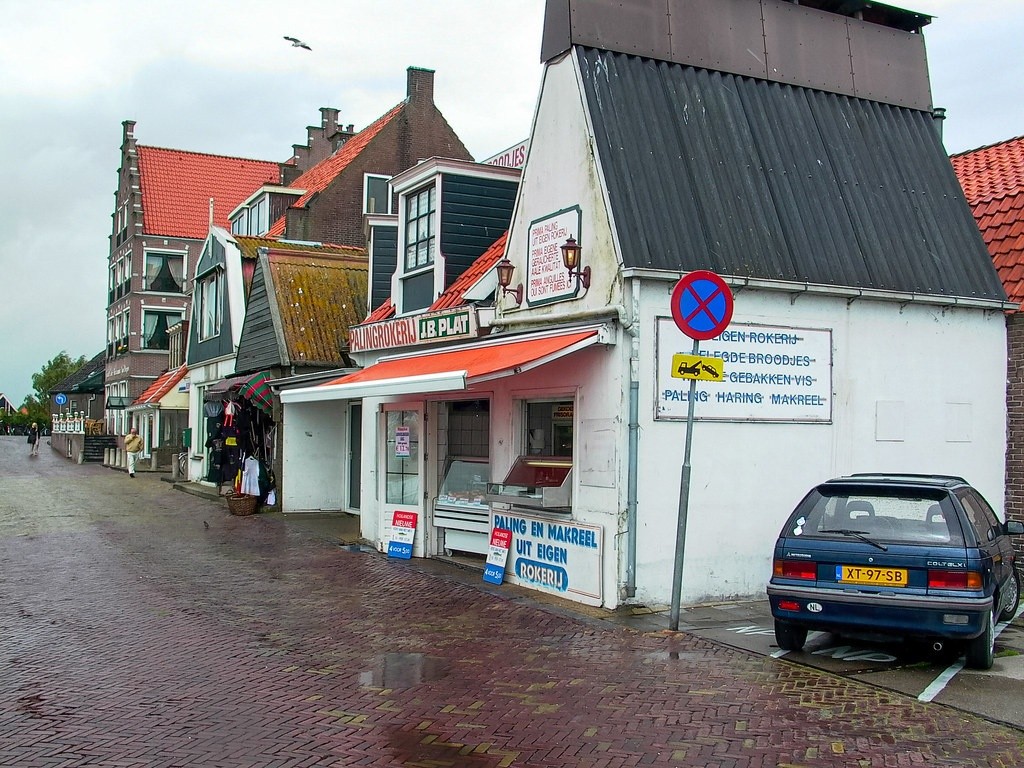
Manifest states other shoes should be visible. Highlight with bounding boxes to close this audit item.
[130,474,135,478]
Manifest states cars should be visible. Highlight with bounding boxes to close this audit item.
[766,470,1024,671]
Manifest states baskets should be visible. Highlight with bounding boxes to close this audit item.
[227,490,257,516]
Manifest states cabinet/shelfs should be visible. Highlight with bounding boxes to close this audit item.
[432,458,494,554]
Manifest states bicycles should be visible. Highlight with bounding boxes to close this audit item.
[0,420,51,437]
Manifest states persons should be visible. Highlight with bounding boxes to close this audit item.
[5,425,11,435]
[124,428,144,479]
[30,422,41,456]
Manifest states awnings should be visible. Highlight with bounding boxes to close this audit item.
[71,370,105,391]
[205,370,273,416]
[279,326,598,403]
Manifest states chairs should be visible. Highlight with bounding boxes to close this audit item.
[843,501,875,531]
[925,504,949,536]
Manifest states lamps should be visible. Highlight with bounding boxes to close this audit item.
[496,255,522,305]
[561,234,591,289]
